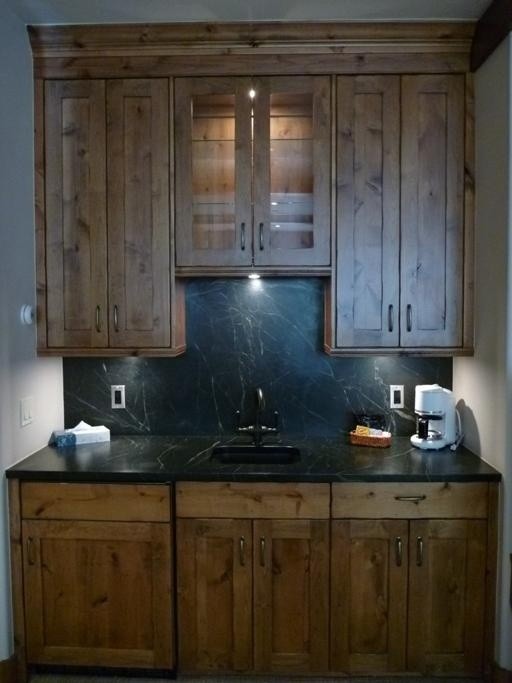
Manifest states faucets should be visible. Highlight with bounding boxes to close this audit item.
[254,387,265,444]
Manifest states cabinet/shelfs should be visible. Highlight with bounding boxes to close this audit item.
[171,476,333,677]
[10,476,172,676]
[334,474,498,680]
[32,69,171,354]
[333,70,474,351]
[173,68,331,271]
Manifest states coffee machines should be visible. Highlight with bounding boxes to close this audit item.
[410,384,458,451]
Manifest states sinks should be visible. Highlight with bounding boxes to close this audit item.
[187,444,302,466]
